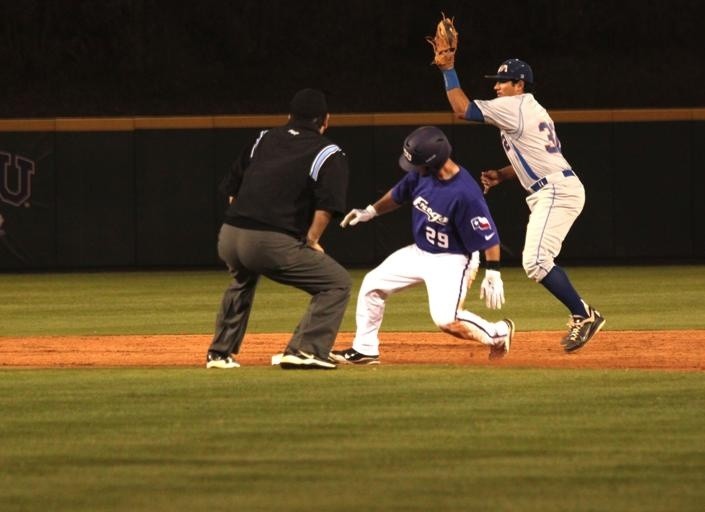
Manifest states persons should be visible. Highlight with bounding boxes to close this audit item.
[328,125,517,369]
[426,13,606,353]
[205,89,352,372]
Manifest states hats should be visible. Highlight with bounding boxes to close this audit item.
[290,85,329,111]
[484,57,534,85]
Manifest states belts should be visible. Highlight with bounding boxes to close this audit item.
[527,170,575,193]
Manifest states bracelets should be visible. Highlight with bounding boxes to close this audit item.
[444,68,460,93]
[485,259,500,272]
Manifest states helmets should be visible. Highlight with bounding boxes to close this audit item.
[398,125,451,173]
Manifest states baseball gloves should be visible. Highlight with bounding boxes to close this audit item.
[425,12,459,70]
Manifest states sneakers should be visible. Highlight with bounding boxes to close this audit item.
[328,346,381,367]
[206,350,241,369]
[489,316,516,361]
[560,306,606,354]
[281,349,337,370]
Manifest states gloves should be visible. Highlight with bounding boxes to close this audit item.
[478,270,505,309]
[340,204,377,228]
